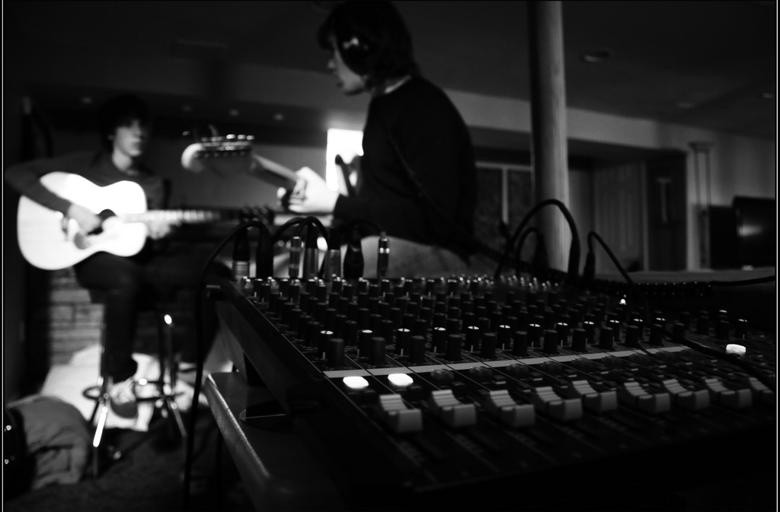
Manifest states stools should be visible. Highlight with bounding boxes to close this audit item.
[81,296,188,491]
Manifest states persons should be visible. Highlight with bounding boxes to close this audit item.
[4,93,185,420]
[277,0,481,280]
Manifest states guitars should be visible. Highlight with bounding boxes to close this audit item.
[17,173,220,271]
[181,133,307,192]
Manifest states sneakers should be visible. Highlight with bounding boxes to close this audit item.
[109,377,137,418]
